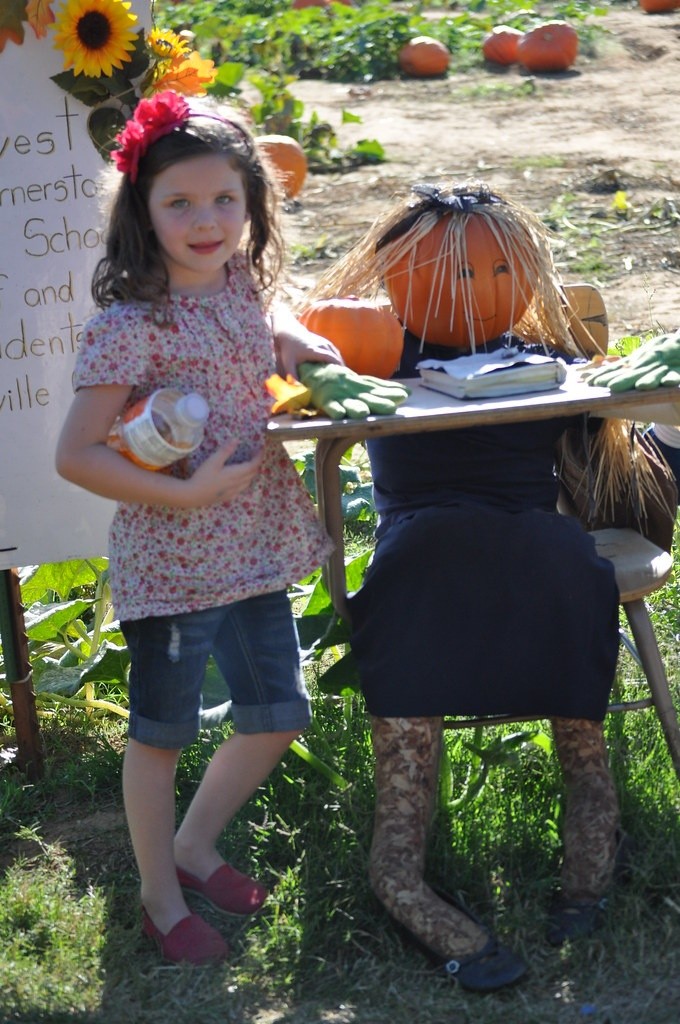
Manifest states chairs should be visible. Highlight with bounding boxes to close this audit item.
[442,282,680,789]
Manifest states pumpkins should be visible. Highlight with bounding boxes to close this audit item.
[292,0,352,12]
[483,25,524,64]
[384,212,542,348]
[254,134,306,200]
[296,297,404,380]
[517,19,578,72]
[638,0,680,13]
[398,36,448,77]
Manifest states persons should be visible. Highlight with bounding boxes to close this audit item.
[55,102,344,970]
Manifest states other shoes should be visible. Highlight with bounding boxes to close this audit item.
[138,901,228,966]
[176,862,269,917]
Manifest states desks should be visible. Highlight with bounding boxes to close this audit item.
[265,355,680,613]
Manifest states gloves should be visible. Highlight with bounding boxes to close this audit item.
[585,330,680,391]
[297,360,410,421]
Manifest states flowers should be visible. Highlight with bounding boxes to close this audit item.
[49,0,221,161]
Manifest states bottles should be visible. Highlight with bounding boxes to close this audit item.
[105,387,209,473]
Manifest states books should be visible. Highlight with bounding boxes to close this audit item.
[418,352,567,400]
